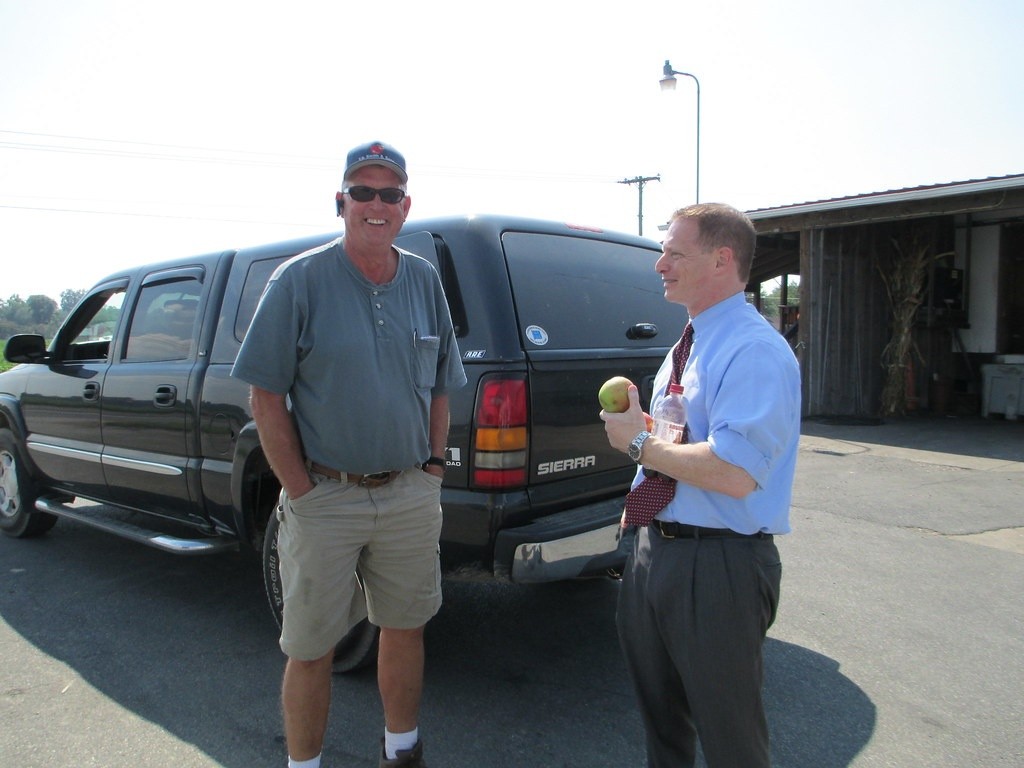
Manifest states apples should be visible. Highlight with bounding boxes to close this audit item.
[598,375,634,413]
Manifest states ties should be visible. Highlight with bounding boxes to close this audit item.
[624,322,694,527]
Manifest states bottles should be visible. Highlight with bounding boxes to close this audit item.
[642,383,687,482]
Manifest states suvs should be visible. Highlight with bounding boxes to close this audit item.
[0,217,688,678]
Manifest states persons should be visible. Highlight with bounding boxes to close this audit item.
[230,142,469,768]
[599,202,801,768]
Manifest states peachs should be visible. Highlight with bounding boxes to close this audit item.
[643,412,653,433]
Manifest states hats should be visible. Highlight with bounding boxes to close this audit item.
[341,142,408,193]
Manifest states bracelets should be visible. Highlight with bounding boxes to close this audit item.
[426,456,447,475]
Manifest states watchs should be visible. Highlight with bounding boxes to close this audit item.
[627,429,653,467]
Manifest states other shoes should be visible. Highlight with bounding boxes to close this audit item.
[378,736,427,768]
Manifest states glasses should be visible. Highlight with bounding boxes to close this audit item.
[341,186,406,204]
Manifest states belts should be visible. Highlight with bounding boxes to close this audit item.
[304,458,402,488]
[650,518,762,539]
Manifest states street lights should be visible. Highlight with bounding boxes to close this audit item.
[660,59,700,205]
